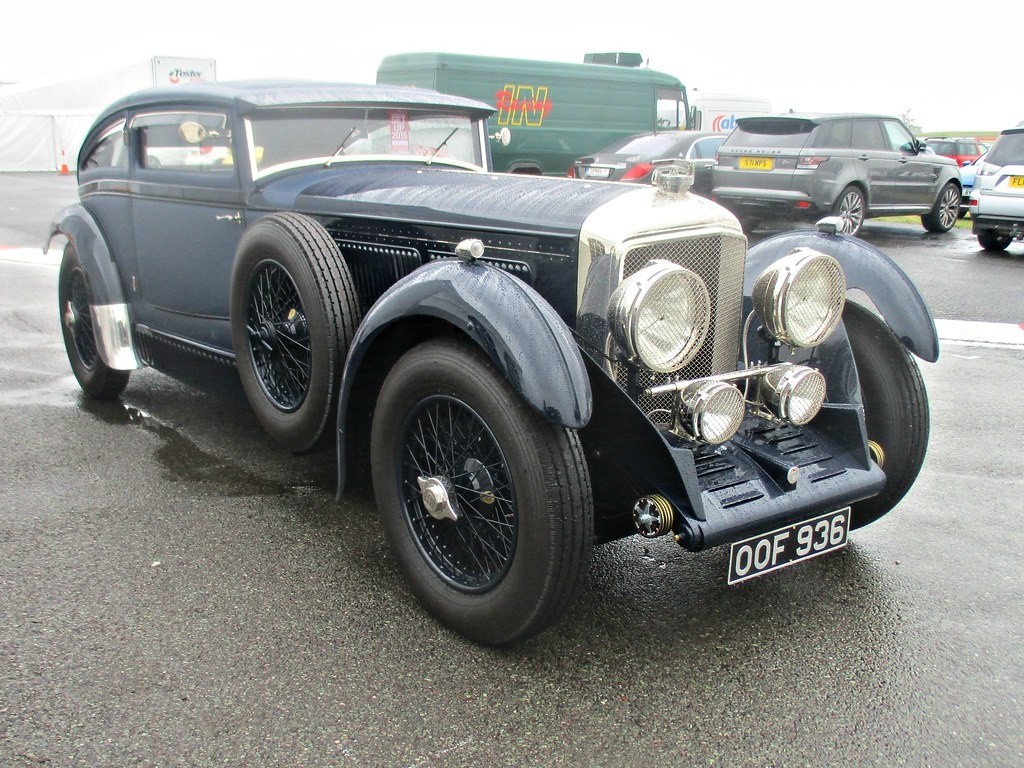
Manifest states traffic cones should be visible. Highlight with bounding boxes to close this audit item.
[60,148,71,176]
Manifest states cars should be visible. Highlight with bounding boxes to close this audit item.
[568,131,731,200]
[957,147,991,219]
[39,77,940,652]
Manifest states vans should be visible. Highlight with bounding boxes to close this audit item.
[376,51,703,179]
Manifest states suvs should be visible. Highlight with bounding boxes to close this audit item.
[923,136,988,170]
[712,108,964,239]
[968,120,1024,253]
